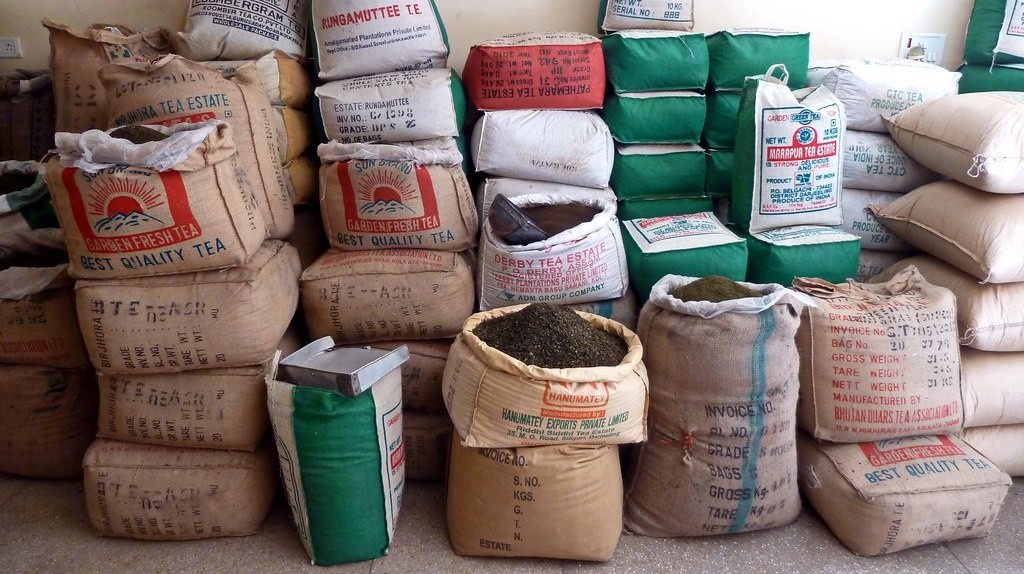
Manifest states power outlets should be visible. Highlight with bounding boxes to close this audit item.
[0,37,23,58]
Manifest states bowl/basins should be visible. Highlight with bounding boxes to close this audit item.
[488,193,551,245]
